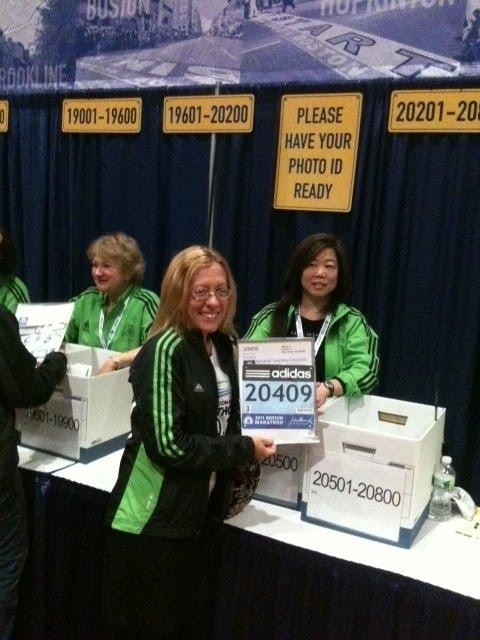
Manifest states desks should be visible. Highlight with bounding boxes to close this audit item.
[12,424,479,632]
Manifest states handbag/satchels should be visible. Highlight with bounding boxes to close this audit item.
[209,460,261,522]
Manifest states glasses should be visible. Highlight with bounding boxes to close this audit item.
[190,288,231,301]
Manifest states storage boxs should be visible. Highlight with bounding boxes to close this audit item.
[222,378,447,549]
[15,341,129,464]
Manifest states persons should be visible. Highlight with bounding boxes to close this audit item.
[102,244,277,640]
[62,231,161,371]
[0,234,67,640]
[241,233,380,416]
[0,228,30,315]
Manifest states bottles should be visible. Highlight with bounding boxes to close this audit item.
[428,455,457,522]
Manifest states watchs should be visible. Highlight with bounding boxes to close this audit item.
[324,380,334,398]
